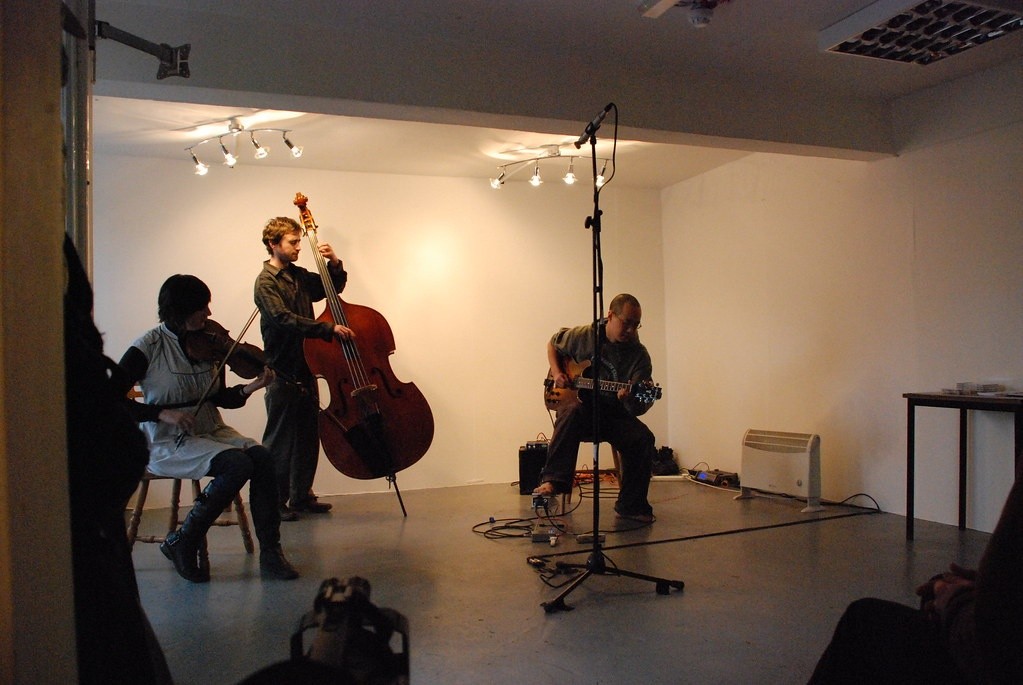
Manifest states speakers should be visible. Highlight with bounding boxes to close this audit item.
[519,446,550,495]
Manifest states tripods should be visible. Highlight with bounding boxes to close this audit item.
[543,133,684,614]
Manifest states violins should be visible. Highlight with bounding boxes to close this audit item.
[178,316,309,397]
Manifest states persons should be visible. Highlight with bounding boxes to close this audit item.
[63,232,175,685]
[109,274,302,583]
[254,217,347,522]
[533,294,655,522]
[806,474,1023,685]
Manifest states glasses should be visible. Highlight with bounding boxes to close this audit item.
[612,312,638,328]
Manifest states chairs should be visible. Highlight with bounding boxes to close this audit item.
[127,383,253,574]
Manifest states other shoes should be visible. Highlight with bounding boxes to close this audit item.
[613,506,653,522]
[533,481,557,496]
[298,496,332,512]
[277,506,298,521]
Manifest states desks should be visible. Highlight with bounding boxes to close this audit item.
[902,392,1023,538]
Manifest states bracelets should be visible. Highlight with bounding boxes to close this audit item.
[241,384,252,396]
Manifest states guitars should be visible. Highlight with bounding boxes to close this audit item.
[544,353,661,411]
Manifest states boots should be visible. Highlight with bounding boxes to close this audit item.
[249,480,300,579]
[159,478,236,582]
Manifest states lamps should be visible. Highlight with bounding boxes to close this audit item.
[489,146,612,189]
[185,120,303,176]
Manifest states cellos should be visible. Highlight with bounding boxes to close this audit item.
[293,193,434,518]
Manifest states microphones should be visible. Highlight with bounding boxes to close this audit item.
[578,105,612,144]
